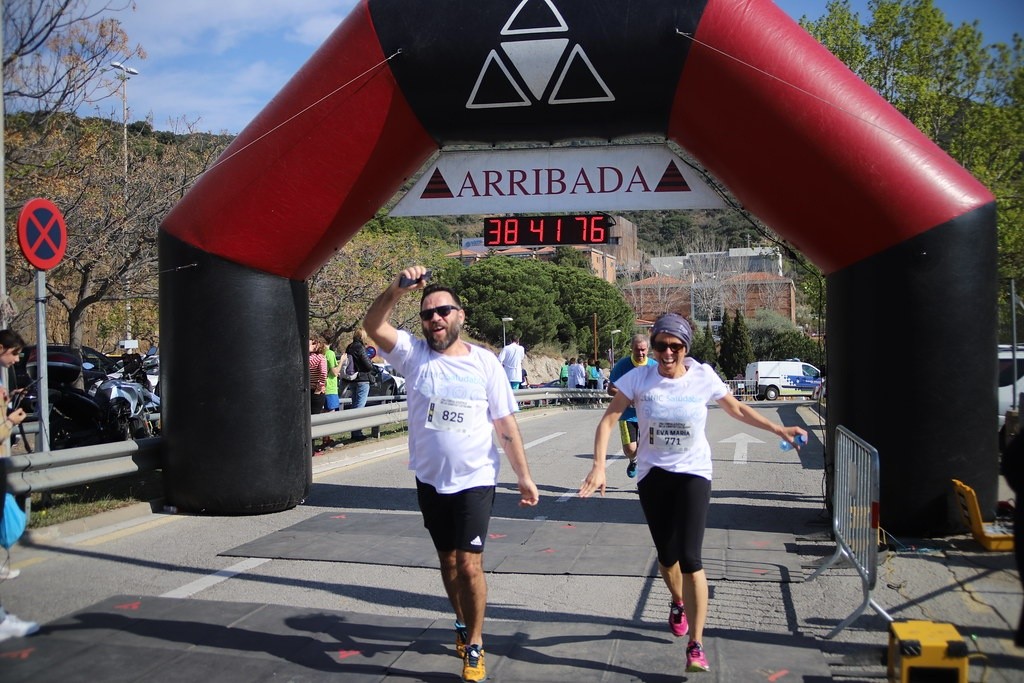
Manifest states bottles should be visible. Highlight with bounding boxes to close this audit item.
[778,434,806,452]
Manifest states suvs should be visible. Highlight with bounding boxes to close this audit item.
[997,343,1024,431]
[10,342,121,388]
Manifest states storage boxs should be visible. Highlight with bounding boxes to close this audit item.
[951,479,1015,551]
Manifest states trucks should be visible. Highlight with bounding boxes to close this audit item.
[745,357,827,401]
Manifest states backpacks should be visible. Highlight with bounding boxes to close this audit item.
[338,342,362,381]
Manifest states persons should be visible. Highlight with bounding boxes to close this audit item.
[607,335,658,477]
[362,265,539,683]
[309,327,377,456]
[0,330,39,642]
[498,335,530,389]
[560,357,609,405]
[578,313,807,672]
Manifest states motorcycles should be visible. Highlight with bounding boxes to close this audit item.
[50,346,160,448]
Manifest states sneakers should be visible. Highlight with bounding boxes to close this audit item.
[626,460,638,477]
[0,614,41,642]
[454,620,468,658]
[669,598,688,637]
[463,645,486,683]
[685,641,710,672]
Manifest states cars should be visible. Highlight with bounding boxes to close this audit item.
[337,355,396,407]
[372,363,407,395]
[89,354,160,396]
[10,363,108,421]
[522,379,561,406]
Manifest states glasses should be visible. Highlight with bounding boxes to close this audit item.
[419,304,461,321]
[652,341,687,352]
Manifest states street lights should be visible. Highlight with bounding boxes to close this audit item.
[110,60,139,352]
[502,317,514,348]
[611,329,622,369]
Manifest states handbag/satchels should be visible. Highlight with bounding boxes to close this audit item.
[0,492,26,548]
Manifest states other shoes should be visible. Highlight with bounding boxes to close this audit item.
[0,566,20,579]
[312,449,325,456]
[326,439,343,448]
[351,434,372,442]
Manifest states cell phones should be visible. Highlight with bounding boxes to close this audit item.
[399,270,432,287]
